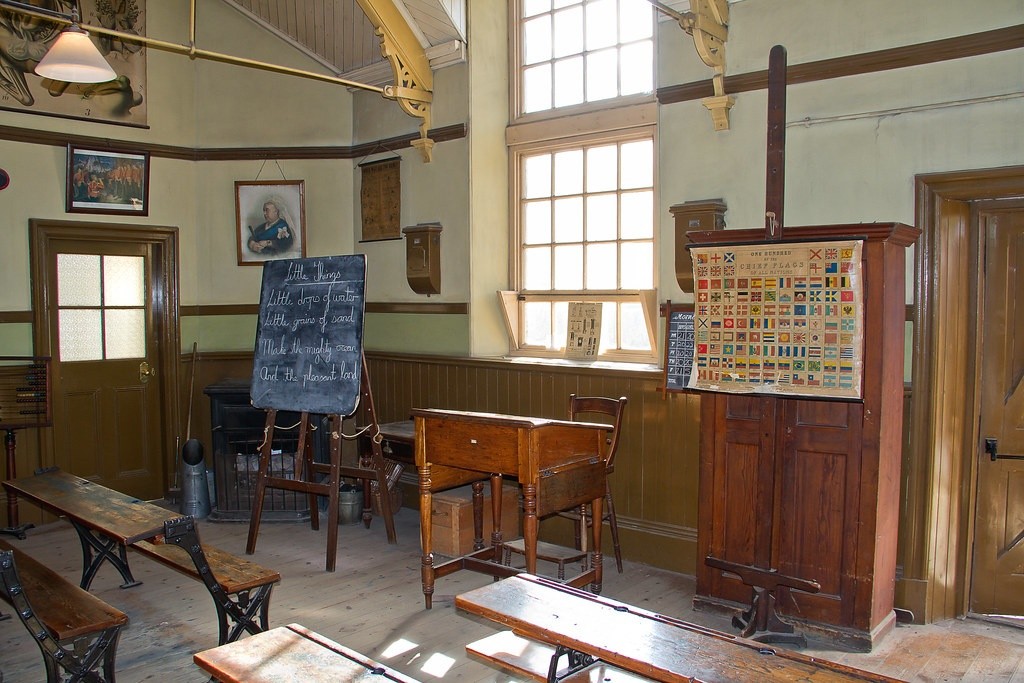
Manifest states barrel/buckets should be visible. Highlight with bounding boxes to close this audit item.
[335,487,363,528]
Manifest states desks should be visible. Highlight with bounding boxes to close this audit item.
[361,420,525,551]
[3,466,909,682]
[408,406,615,611]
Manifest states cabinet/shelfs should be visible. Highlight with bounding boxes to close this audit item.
[681,221,929,655]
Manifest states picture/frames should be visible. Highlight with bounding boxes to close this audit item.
[65,141,151,218]
[234,178,306,267]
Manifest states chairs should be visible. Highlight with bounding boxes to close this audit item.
[534,390,628,576]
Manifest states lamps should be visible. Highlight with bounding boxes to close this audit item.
[36,0,118,86]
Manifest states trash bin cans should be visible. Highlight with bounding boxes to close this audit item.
[179,438,212,521]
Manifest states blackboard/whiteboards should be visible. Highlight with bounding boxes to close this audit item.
[249,253,368,418]
